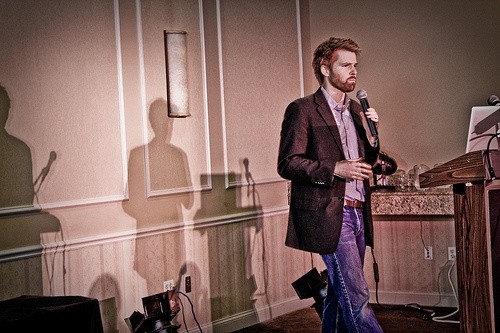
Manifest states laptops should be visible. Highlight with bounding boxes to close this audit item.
[465,106,500,153]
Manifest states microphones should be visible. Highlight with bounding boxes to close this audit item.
[355,90,378,137]
[487,94,500,106]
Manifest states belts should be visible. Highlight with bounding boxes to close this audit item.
[344,199,366,209]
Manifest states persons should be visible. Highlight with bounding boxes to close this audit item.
[276,38,386,333]
